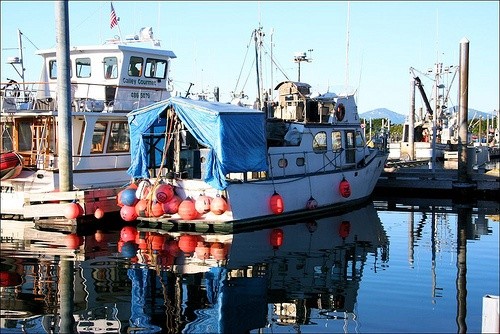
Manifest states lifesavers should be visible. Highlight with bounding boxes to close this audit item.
[336,104,345,121]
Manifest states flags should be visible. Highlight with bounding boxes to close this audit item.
[110,2,118,29]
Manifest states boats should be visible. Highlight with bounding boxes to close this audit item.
[119,93,391,227]
[0,1,177,227]
[232,1,500,190]
